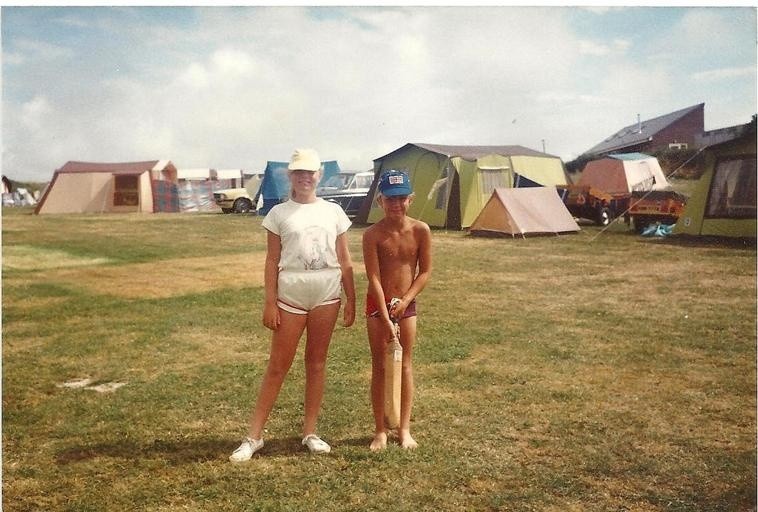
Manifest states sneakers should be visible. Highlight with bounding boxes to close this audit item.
[301,434,331,454]
[228,436,264,462]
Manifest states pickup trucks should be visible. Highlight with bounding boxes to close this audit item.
[628,191,685,232]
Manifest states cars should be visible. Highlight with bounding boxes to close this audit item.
[213,187,254,213]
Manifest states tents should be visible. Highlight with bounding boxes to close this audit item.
[571,151,673,196]
[31,158,343,220]
[354,140,574,236]
[662,134,757,247]
[467,184,584,238]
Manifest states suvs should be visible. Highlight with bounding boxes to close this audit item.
[279,172,374,214]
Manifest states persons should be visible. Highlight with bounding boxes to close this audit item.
[359,166,432,454]
[223,148,357,465]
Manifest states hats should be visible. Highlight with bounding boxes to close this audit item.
[288,149,321,172]
[378,170,412,196]
